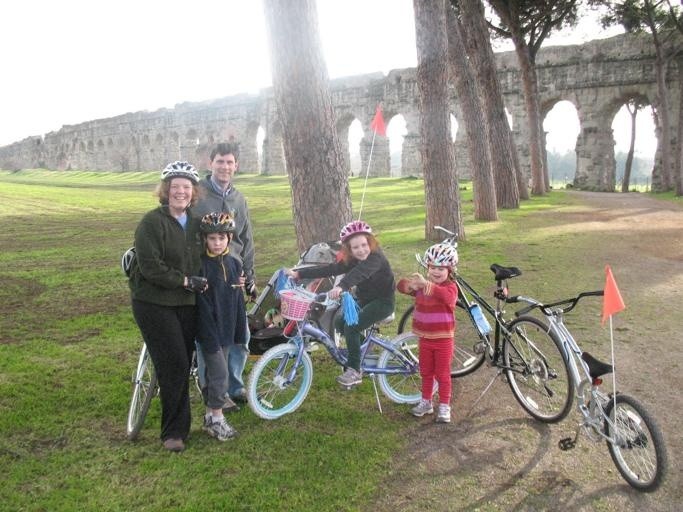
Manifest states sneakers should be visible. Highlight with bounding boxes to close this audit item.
[436,403,451,423]
[163,388,254,451]
[335,367,362,386]
[412,400,433,416]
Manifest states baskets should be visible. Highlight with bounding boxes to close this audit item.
[279,289,313,322]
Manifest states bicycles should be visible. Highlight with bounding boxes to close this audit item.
[121,245,337,441]
[246,272,439,420]
[397,225,667,493]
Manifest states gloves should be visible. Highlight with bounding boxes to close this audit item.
[185,275,207,294]
[246,281,259,296]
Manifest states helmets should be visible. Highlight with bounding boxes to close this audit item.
[423,243,458,266]
[161,160,200,184]
[199,211,236,233]
[122,246,136,276]
[339,221,371,241]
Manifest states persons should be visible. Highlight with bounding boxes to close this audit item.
[397,243,459,424]
[128,160,248,452]
[188,140,260,412]
[193,210,250,443]
[285,220,396,387]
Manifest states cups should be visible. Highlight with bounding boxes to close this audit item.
[469,301,492,336]
